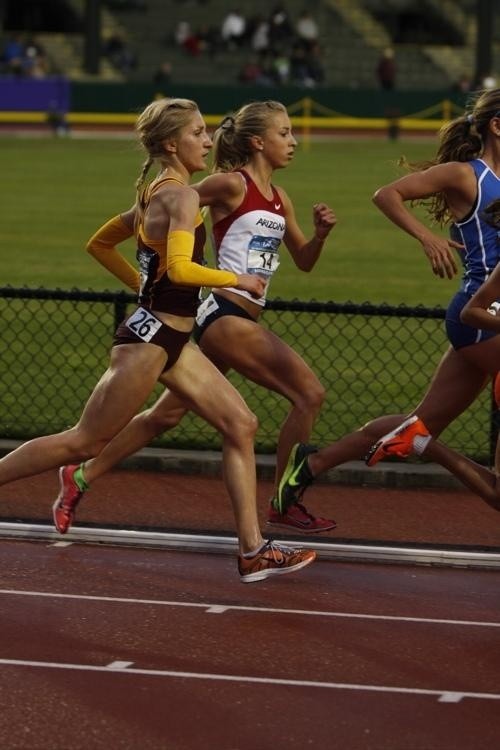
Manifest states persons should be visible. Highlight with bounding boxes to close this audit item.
[51,96,338,536]
[365,199,500,516]
[1,92,319,585]
[268,85,500,521]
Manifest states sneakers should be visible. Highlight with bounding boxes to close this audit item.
[265,499,337,533]
[52,463,83,535]
[274,441,318,518]
[237,538,317,584]
[364,414,430,469]
